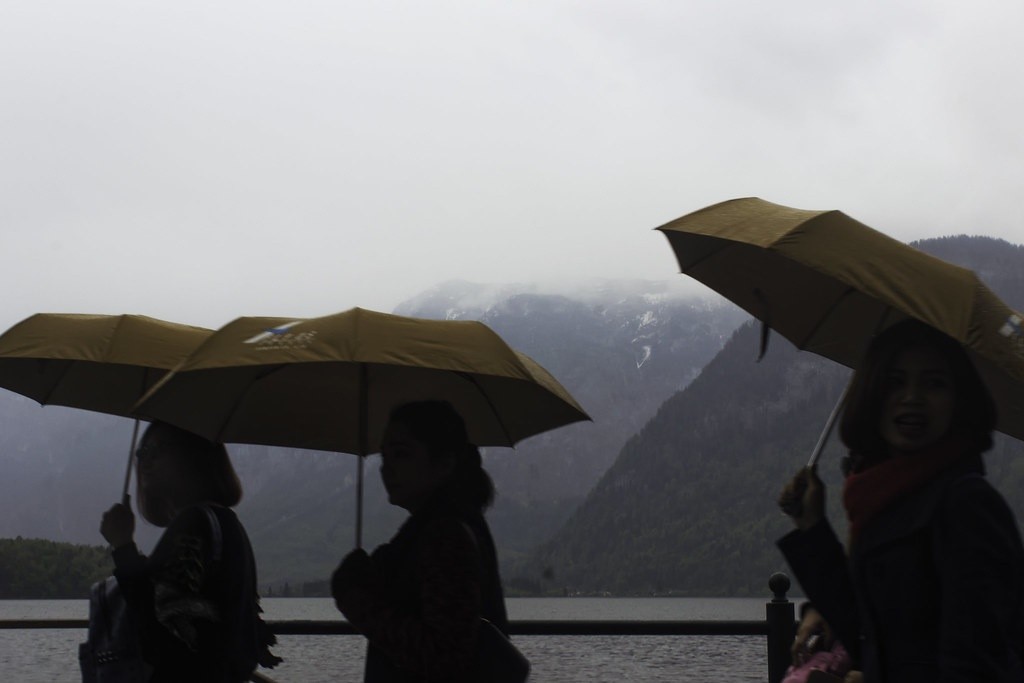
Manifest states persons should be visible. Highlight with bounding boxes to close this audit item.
[775,319,1024,683]
[330,402,511,683]
[100,423,258,683]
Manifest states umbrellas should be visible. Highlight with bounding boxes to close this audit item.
[652,197,1024,519]
[0,313,213,501]
[129,308,594,549]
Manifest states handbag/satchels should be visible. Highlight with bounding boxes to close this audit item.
[80,576,142,683]
[471,618,529,683]
